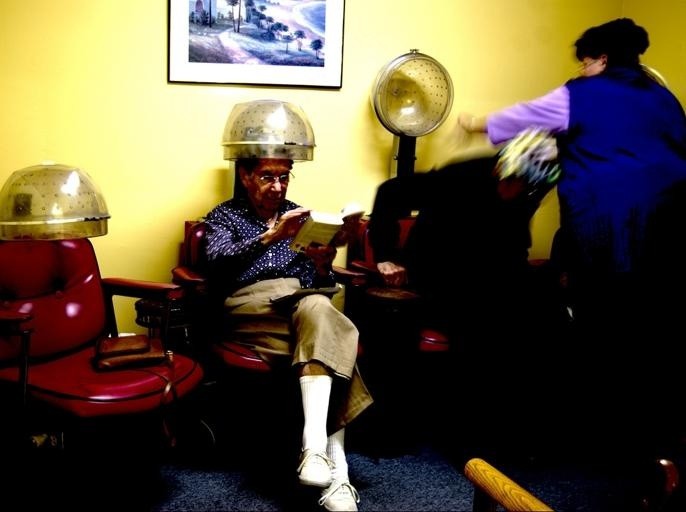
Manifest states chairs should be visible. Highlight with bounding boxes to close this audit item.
[345,212,451,355]
[0,230,206,479]
[459,455,551,511]
[163,220,367,465]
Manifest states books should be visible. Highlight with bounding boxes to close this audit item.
[290,202,365,254]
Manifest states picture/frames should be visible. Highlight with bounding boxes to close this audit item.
[165,0,346,91]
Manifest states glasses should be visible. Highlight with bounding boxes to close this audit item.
[248,173,288,185]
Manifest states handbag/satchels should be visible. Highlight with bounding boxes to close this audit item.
[91,331,169,375]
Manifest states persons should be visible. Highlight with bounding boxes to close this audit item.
[461,19,685,512]
[197,130,376,512]
[368,135,561,466]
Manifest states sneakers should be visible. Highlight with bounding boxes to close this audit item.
[316,482,359,512]
[294,447,334,488]
